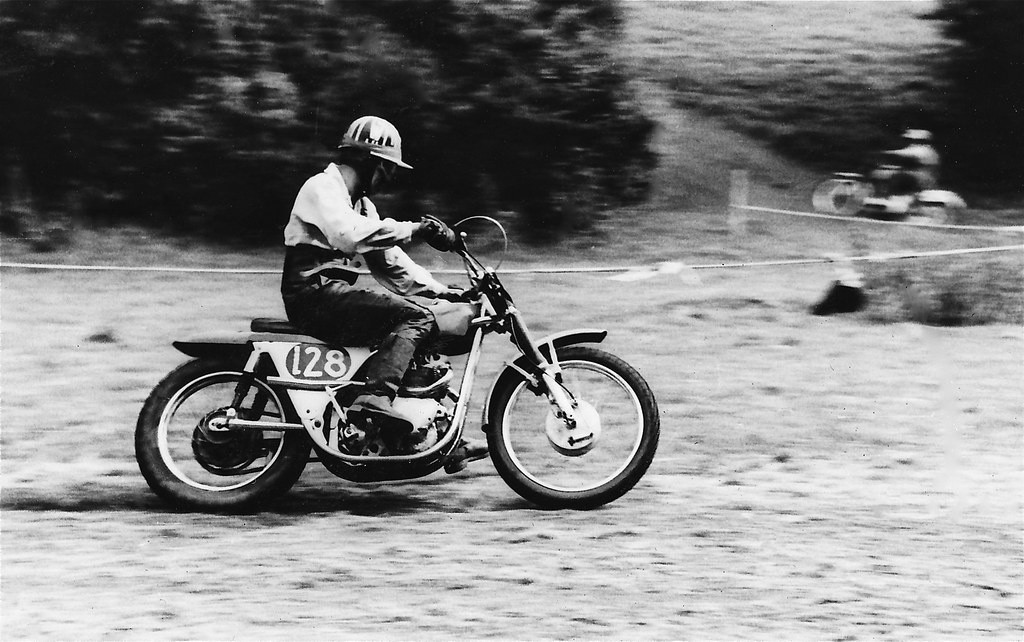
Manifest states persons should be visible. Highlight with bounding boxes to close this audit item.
[280,116,459,438]
[872,129,939,198]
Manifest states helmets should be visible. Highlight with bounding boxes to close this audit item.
[338,116,413,171]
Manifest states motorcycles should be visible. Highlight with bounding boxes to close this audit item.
[809,157,962,234]
[135,214,659,512]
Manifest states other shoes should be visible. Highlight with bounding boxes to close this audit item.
[347,391,414,437]
[444,435,489,474]
